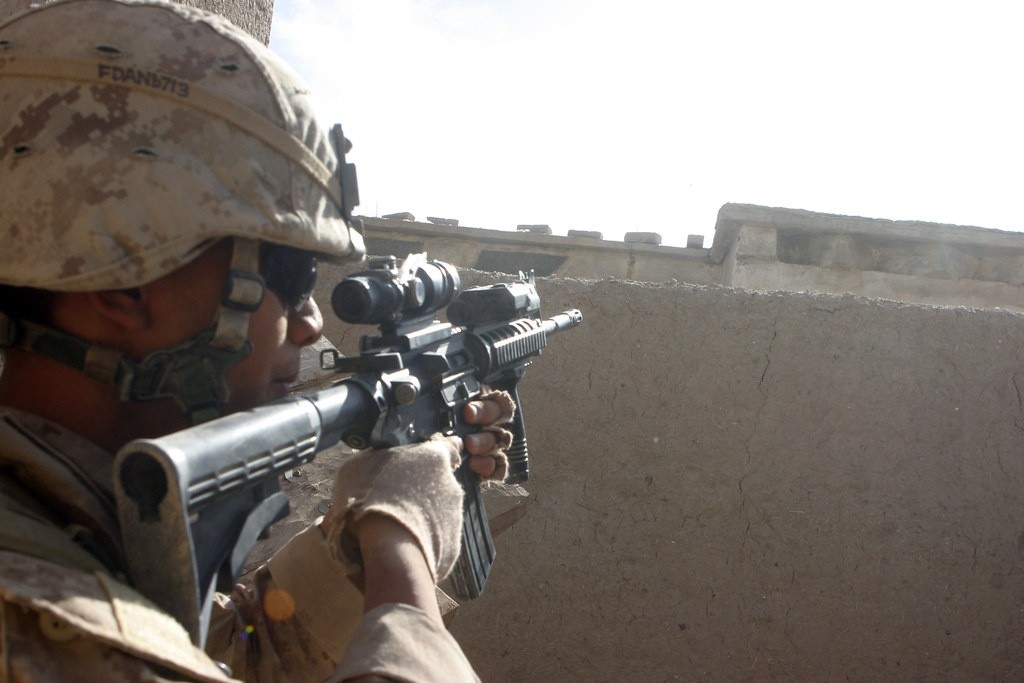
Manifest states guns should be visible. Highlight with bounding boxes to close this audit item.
[115,256,585,647]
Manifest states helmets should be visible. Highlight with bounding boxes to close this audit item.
[0,0,367,292]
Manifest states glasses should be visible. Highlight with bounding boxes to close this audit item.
[258,240,319,313]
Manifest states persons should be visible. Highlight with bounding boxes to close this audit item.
[1,0,515,683]
